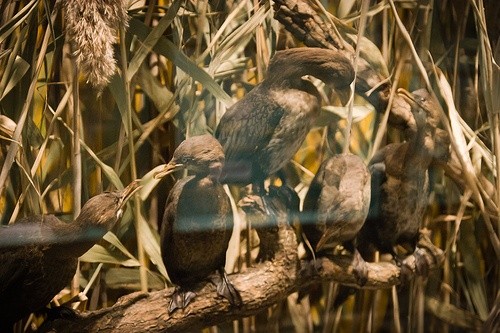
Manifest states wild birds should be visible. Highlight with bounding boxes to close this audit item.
[215,45,357,216]
[304,104,372,280]
[154,135,244,315]
[354,88,451,283]
[1,179,141,333]
